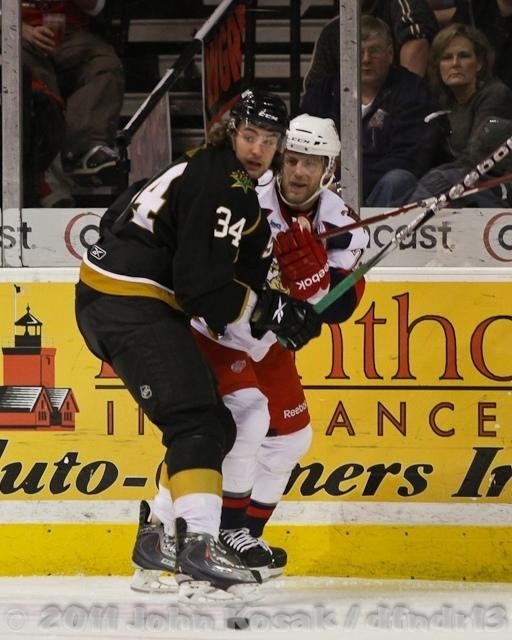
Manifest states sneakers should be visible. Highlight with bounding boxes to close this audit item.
[258,539,287,568]
[67,145,123,186]
[219,528,273,567]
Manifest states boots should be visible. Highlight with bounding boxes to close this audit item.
[132,499,178,571]
[174,516,262,587]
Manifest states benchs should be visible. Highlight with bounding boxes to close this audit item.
[73,0,337,196]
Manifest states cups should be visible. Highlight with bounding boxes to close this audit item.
[41,13,65,56]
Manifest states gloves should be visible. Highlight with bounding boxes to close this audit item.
[249,290,321,350]
[272,222,330,300]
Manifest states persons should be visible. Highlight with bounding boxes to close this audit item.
[72,87,324,589]
[306,12,440,211]
[182,111,373,578]
[362,21,512,208]
[371,0,512,77]
[21,0,136,212]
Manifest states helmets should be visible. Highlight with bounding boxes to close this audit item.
[280,113,341,173]
[228,90,289,153]
